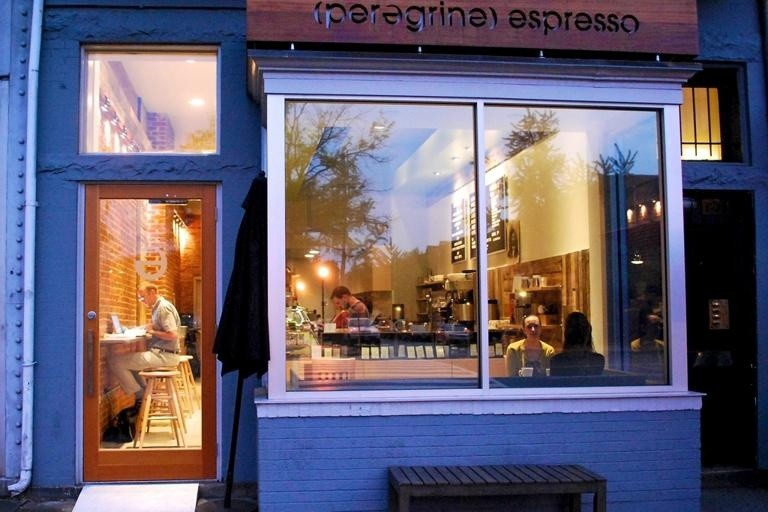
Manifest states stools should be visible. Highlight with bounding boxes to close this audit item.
[137,352,195,446]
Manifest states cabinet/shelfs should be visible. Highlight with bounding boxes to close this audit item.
[494,254,566,331]
[414,273,477,323]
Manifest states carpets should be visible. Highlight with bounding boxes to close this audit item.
[72,483,200,512]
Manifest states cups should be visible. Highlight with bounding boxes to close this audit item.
[517,367,534,378]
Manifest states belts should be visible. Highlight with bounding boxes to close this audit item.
[162,349,178,354]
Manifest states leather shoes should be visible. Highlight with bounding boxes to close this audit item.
[130,399,142,417]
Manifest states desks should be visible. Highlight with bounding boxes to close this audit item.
[287,354,509,392]
[102,326,154,343]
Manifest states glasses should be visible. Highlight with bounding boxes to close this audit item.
[138,296,145,302]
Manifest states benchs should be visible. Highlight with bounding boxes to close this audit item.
[384,465,608,511]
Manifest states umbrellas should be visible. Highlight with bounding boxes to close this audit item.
[210,171,270,509]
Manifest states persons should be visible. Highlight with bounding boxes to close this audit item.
[506,312,605,376]
[629,292,664,373]
[109,283,181,418]
[329,286,369,328]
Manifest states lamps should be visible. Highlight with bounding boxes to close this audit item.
[101,95,136,152]
[629,166,645,266]
